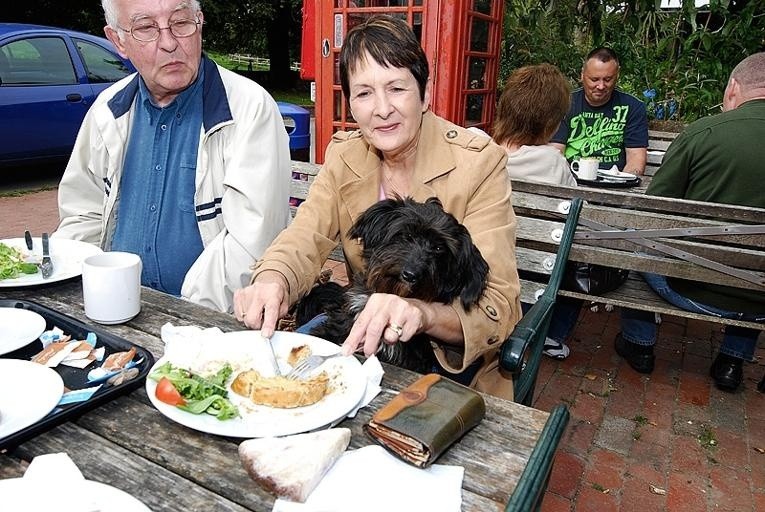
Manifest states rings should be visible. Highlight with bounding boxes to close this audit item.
[389,324,402,335]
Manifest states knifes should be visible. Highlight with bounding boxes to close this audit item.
[40,230,56,280]
[265,337,282,378]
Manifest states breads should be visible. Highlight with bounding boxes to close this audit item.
[231,345,328,409]
[238,427,351,504]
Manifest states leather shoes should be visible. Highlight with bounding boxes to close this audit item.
[614,330,656,374]
[709,354,743,393]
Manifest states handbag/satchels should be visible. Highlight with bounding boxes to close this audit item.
[575,259,630,295]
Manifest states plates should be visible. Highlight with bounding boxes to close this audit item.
[1,238,108,287]
[1,358,65,441]
[0,306,48,355]
[598,170,638,180]
[141,328,367,436]
[2,476,156,512]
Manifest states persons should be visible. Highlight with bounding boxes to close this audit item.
[614,51,765,393]
[48,0,292,313]
[491,62,584,360]
[234,13,523,403]
[548,46,649,175]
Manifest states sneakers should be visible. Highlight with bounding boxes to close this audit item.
[541,336,571,361]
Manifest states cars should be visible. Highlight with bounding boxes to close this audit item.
[0,22,140,165]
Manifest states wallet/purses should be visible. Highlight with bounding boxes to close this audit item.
[362,373,486,468]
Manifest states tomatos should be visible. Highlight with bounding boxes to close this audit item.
[155,376,186,407]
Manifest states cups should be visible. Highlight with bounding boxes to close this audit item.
[83,251,144,326]
[571,157,599,180]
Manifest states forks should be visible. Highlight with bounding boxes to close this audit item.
[286,340,371,380]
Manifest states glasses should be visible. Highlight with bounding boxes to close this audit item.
[117,17,201,42]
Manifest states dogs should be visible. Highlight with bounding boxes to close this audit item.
[294,190,493,388]
